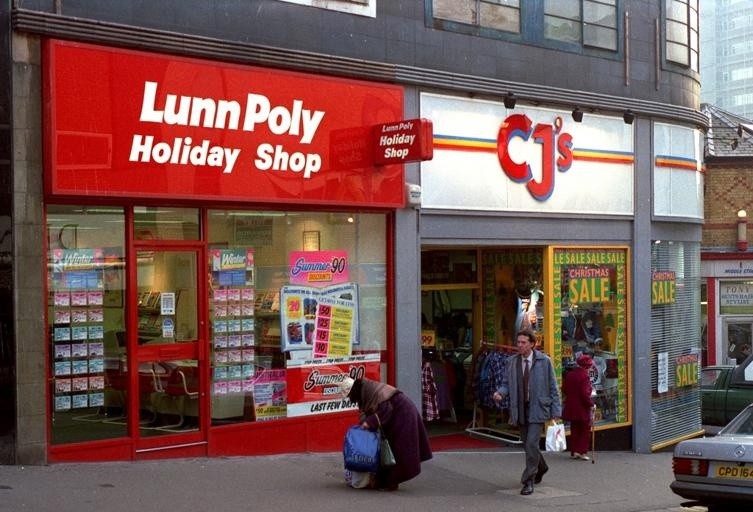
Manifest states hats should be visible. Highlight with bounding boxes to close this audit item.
[577,355,592,369]
[340,378,355,397]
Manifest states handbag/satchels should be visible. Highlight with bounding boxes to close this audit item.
[545,418,567,453]
[344,467,372,489]
[342,423,381,473]
[381,436,395,468]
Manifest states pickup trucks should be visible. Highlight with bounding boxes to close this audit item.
[700,351,752,427]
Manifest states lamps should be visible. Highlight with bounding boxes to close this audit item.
[707,123,743,137]
[589,106,635,125]
[736,210,749,250]
[467,90,516,110]
[531,100,582,124]
[703,136,738,151]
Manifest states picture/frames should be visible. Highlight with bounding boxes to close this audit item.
[233,216,274,247]
[301,231,319,251]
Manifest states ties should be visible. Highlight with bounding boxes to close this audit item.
[522,357,531,403]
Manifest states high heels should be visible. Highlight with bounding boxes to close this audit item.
[574,452,590,460]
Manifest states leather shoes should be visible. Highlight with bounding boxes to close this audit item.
[521,479,533,495]
[534,464,549,484]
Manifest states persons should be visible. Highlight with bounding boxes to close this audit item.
[345,376,432,489]
[732,342,752,365]
[490,327,564,495]
[558,353,596,460]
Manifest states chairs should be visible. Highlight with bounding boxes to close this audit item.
[72,359,199,433]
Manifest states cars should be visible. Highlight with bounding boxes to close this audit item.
[667,402,752,511]
